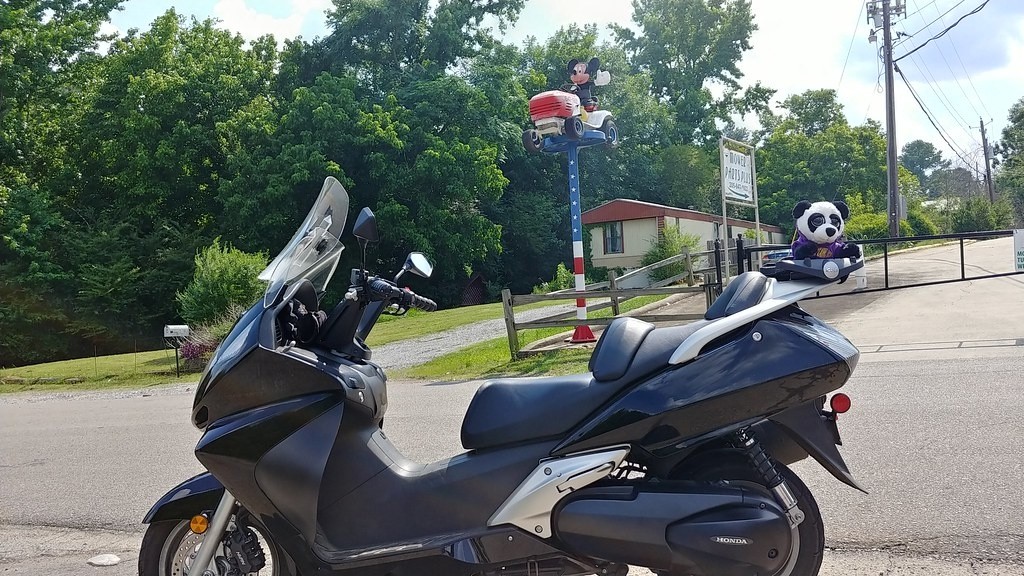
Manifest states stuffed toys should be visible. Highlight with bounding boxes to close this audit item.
[791,200,862,260]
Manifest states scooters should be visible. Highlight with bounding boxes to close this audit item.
[138,176,871,575]
